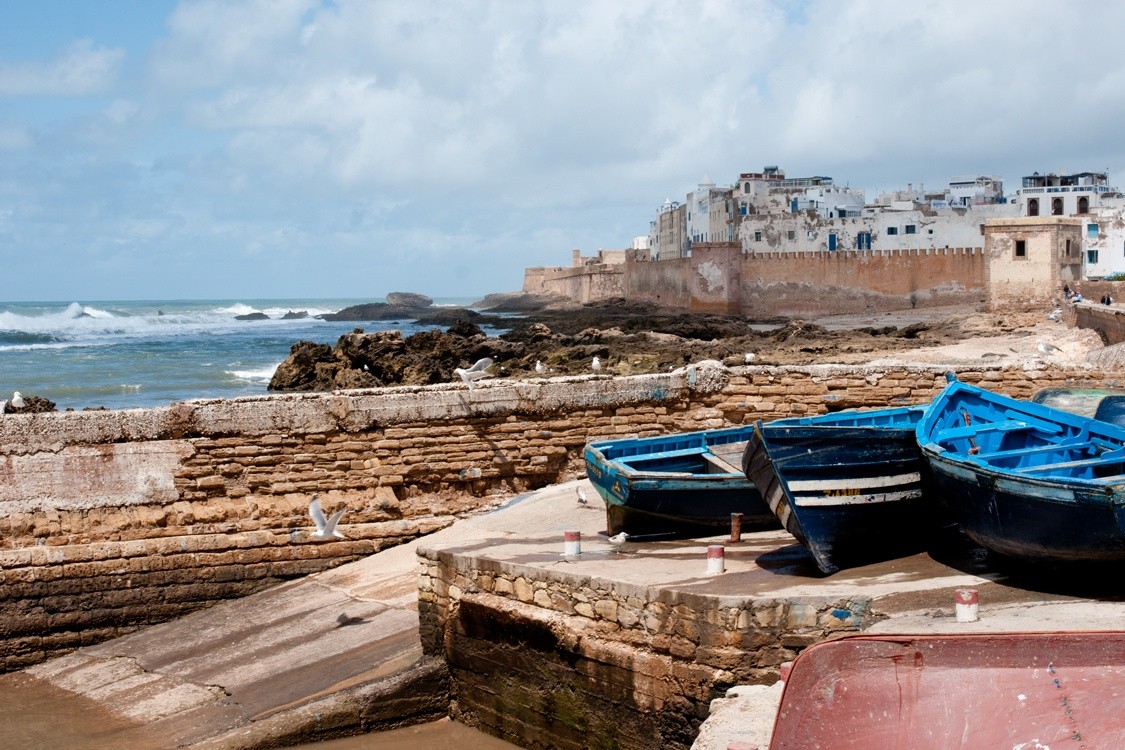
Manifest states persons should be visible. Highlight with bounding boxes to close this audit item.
[1064,283,1082,302]
[1100,291,1111,305]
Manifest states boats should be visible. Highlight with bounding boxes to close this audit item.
[583,404,931,520]
[915,368,1125,575]
[742,420,926,573]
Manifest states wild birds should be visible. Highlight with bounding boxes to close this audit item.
[364,364,369,371]
[535,360,546,378]
[591,357,601,375]
[309,493,349,539]
[608,532,630,553]
[12,392,26,413]
[454,358,493,389]
[576,484,587,503]
[1036,341,1063,361]
